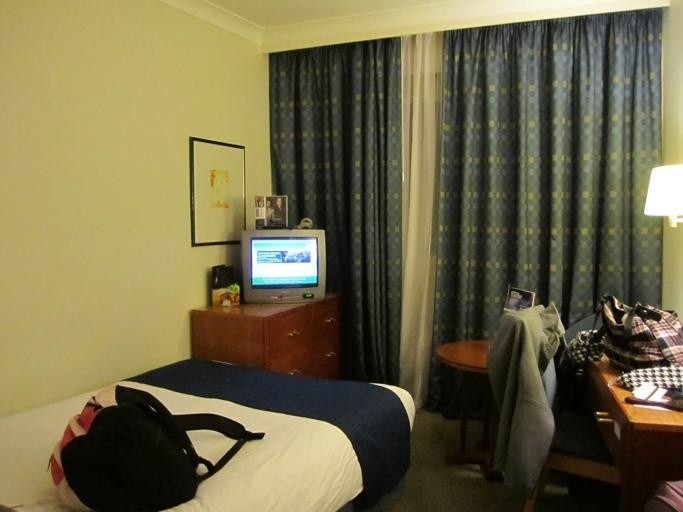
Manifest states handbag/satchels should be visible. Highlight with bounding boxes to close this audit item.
[594,293,683,372]
[558,329,607,379]
[46,384,200,511]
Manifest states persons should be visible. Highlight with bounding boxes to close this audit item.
[267,200,275,224]
[269,197,286,228]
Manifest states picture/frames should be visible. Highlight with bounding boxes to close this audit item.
[189,136,247,247]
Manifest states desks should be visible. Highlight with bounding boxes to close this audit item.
[432,341,499,470]
[588,353,683,511]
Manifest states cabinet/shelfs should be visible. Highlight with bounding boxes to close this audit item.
[192,293,341,378]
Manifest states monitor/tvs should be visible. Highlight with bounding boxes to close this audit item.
[239,229,327,303]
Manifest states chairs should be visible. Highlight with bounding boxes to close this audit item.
[523,428,622,511]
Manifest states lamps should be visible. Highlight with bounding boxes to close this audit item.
[643,164,683,228]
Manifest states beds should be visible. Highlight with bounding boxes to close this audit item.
[0,359,411,511]
